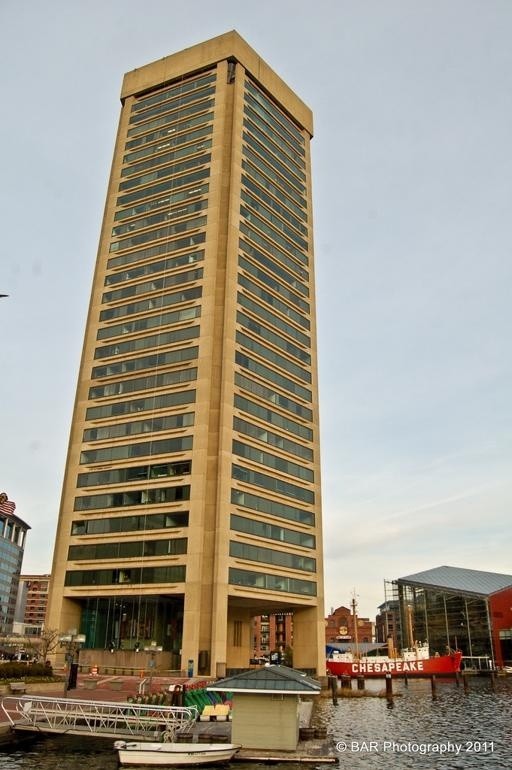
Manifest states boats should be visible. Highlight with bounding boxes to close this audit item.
[114,740,242,766]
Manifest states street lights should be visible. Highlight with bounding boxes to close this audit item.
[143,641,163,692]
[57,628,86,698]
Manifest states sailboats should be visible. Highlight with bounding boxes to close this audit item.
[326,599,464,678]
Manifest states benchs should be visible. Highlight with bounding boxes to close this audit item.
[9,682,27,694]
